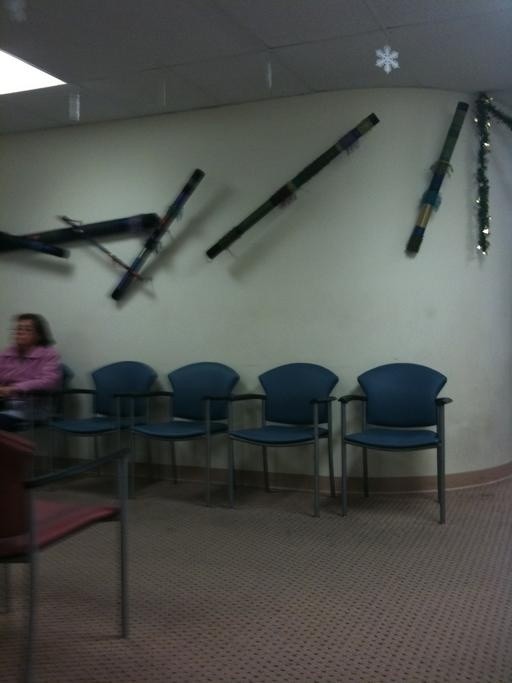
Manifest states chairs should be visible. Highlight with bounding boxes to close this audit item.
[227,362,338,519]
[336,363,454,523]
[51,361,159,491]
[1,430,130,680]
[0,361,76,485]
[128,361,242,499]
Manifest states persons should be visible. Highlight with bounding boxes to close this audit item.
[1,312,66,433]
